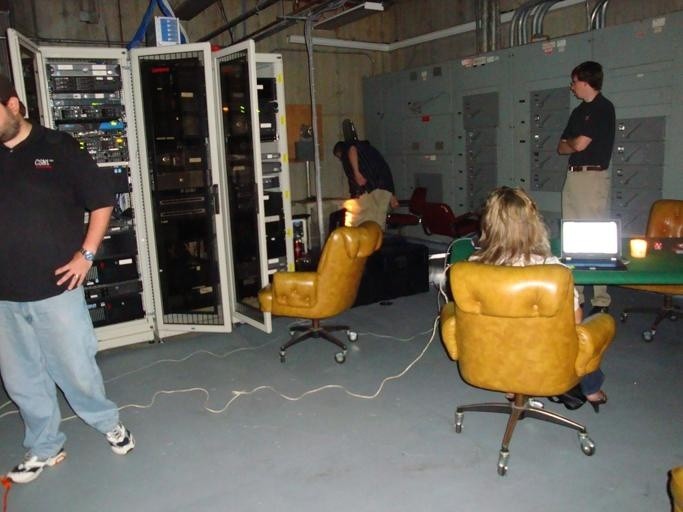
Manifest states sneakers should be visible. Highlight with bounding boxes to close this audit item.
[104,418,135,456]
[5,443,68,485]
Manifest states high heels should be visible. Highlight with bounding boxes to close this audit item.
[589,390,608,415]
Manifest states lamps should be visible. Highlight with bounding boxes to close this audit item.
[312,1,384,31]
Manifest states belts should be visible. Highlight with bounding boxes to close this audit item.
[568,164,609,172]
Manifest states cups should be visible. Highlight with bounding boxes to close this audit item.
[630,239,646,259]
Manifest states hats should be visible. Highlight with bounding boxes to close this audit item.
[0,73,26,117]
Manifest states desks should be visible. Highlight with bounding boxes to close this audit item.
[449,238,683,322]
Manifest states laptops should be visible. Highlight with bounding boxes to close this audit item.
[558,218,621,269]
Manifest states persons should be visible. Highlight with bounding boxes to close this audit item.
[332,140,400,235]
[466,184,608,414]
[557,60,616,317]
[0,73,137,484]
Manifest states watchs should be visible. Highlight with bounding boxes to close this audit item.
[78,245,98,263]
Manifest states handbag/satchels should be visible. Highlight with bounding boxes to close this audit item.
[547,384,587,411]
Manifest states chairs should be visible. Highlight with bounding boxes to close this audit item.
[385,187,427,237]
[258,221,383,363]
[666,466,683,512]
[329,209,428,308]
[440,261,617,476]
[620,200,683,342]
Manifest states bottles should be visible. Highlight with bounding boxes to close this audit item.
[295,237,304,261]
